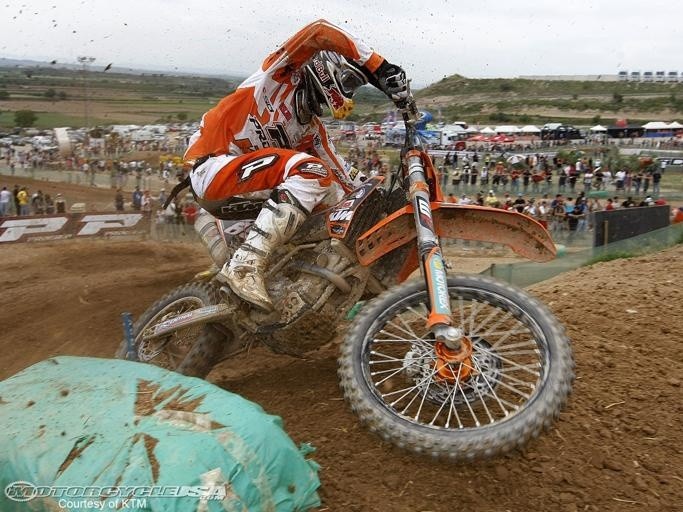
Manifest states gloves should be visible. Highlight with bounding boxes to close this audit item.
[371,58,409,103]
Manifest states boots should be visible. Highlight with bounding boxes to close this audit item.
[215,190,310,314]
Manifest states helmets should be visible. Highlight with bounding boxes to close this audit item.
[302,49,370,121]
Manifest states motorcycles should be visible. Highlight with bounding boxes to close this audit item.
[113,68,576,465]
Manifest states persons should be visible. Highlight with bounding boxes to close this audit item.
[161,18,412,313]
[0,121,199,240]
